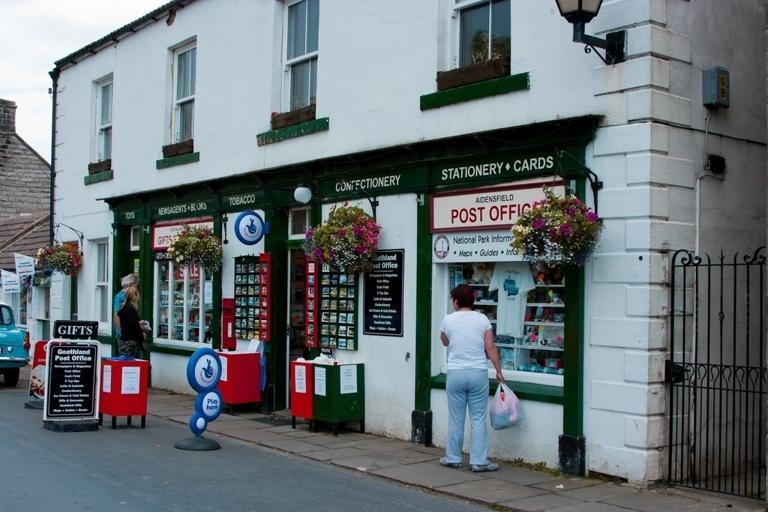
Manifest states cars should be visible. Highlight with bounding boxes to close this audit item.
[0,301,29,388]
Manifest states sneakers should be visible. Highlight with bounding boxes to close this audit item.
[440,456,463,468]
[472,461,500,472]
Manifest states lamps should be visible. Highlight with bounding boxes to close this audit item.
[556,0,628,65]
[292,181,316,203]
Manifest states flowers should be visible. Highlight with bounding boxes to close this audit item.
[508,182,604,273]
[167,223,223,275]
[39,243,82,277]
[300,202,383,276]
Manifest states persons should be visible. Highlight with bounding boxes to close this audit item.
[112,273,138,350]
[117,285,147,359]
[439,284,504,472]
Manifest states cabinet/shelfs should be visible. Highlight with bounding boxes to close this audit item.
[153,259,214,349]
[444,262,564,387]
